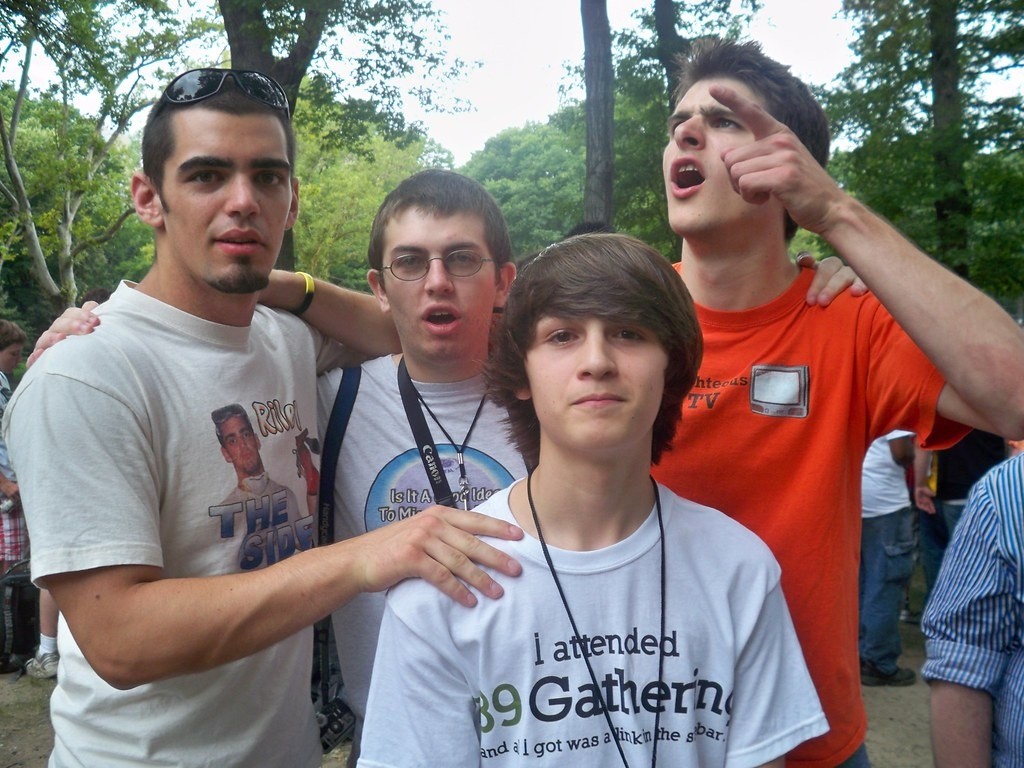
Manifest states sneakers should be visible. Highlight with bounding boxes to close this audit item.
[25,650,60,679]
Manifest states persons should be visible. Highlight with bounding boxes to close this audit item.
[0,36,1024,768]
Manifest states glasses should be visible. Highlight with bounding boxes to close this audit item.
[379,250,502,282]
[150,68,290,123]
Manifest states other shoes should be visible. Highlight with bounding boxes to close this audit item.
[859,662,915,688]
[0,663,21,674]
[899,609,923,624]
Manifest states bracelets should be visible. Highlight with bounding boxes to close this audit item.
[291,271,313,316]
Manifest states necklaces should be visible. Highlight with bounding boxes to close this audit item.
[523,471,666,768]
[399,367,490,512]
